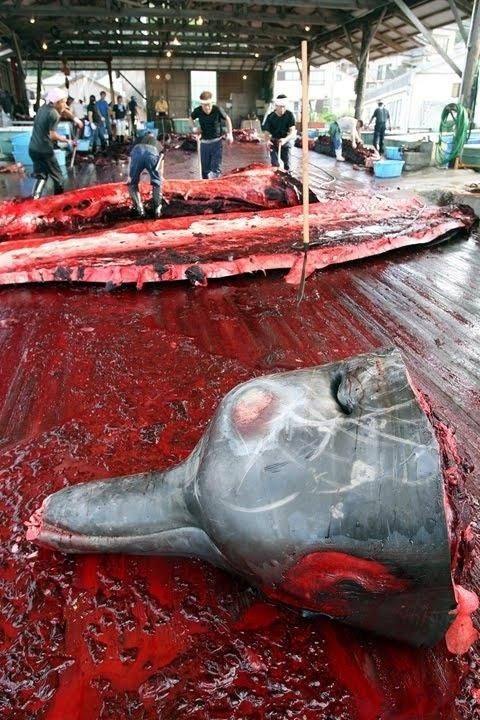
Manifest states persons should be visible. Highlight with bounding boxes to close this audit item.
[365,101,392,155]
[13,98,32,121]
[188,91,233,180]
[64,92,141,156]
[154,96,169,117]
[28,88,85,201]
[263,95,297,173]
[125,130,167,219]
[328,116,364,161]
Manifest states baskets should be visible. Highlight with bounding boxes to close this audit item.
[373,161,405,177]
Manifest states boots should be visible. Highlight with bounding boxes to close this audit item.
[153,187,165,218]
[129,185,146,217]
[334,149,345,162]
[30,178,46,199]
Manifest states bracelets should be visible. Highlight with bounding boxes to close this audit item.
[226,133,232,137]
[193,127,198,131]
